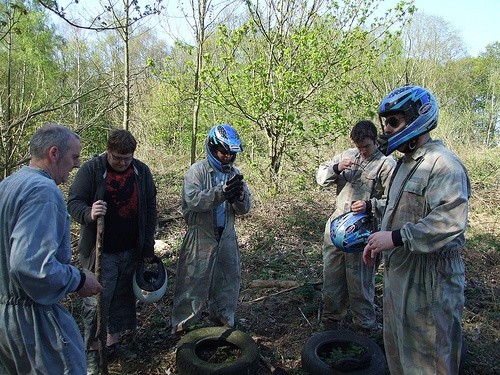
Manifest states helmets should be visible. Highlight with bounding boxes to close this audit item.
[205,125,244,173]
[329,211,375,253]
[131,255,168,305]
[375,86,439,157]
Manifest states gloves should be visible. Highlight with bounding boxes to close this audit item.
[222,174,244,198]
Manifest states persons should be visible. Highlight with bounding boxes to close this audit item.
[171,123,251,337]
[0,124,104,375]
[67,129,157,375]
[317,121,397,335]
[362,85,471,375]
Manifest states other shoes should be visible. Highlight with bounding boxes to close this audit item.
[106,342,137,360]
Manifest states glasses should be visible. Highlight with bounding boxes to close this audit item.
[385,117,405,128]
[343,163,365,184]
[112,153,133,161]
